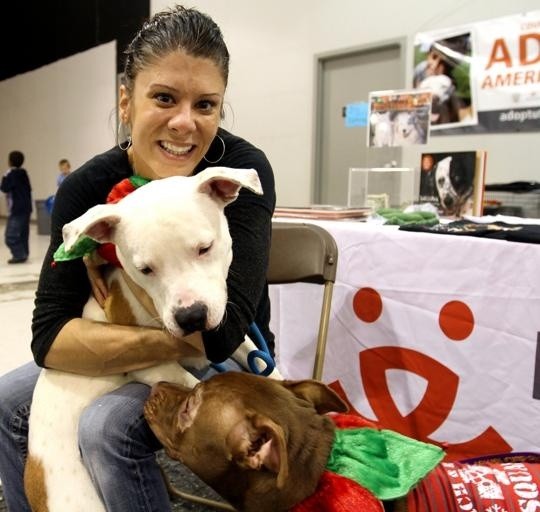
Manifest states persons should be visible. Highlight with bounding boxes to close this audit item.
[412,35,467,125]
[0,4,277,512]
[56,158,72,187]
[0,149,33,264]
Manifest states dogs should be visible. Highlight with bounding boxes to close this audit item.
[22,166,263,512]
[143,371,384,512]
[392,111,425,144]
[414,73,456,124]
[425,153,475,216]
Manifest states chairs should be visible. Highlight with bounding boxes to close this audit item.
[268,222,338,378]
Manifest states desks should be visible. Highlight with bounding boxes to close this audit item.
[269,216,540,457]
